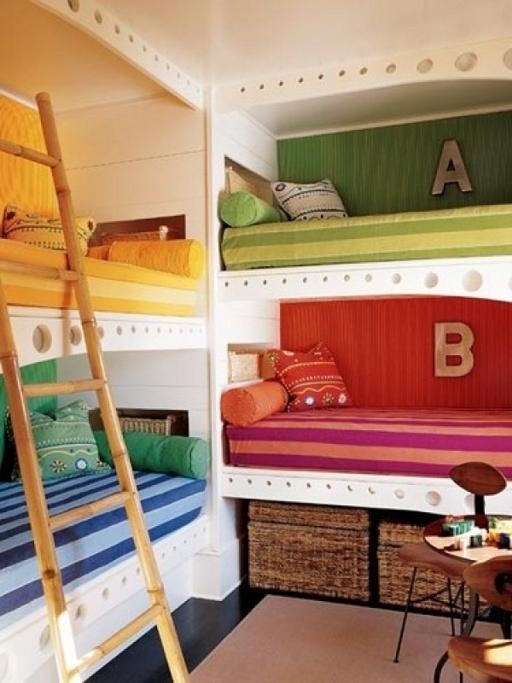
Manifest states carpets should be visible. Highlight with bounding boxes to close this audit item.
[190,594,505,683]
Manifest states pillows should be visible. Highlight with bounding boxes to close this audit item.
[270,178,349,222]
[108,239,205,280]
[266,340,355,412]
[5,399,113,484]
[220,192,288,228]
[3,205,96,257]
[221,378,292,426]
[93,430,211,481]
[87,245,108,260]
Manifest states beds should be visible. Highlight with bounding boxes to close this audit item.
[222,153,512,514]
[0,0,219,682]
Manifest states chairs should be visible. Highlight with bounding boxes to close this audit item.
[392,461,507,662]
[446,555,512,683]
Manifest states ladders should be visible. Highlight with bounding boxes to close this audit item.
[0,91,191,683]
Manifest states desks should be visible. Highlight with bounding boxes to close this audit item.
[424,515,512,683]
